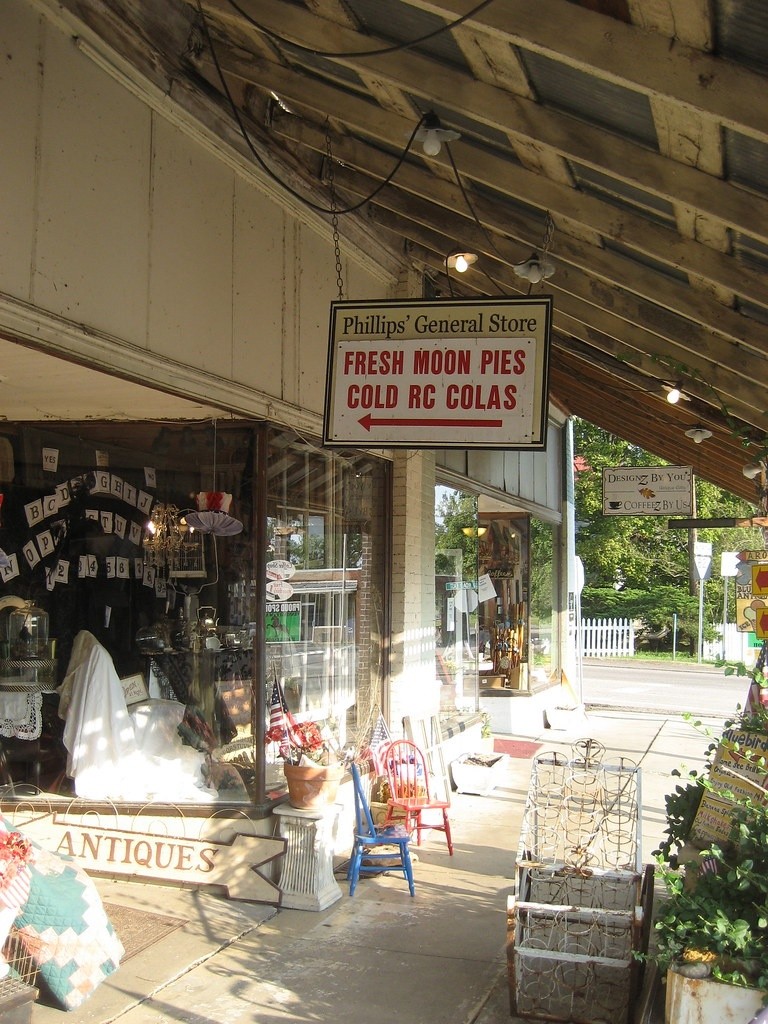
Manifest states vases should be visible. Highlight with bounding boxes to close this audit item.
[452,748,509,797]
[284,757,344,810]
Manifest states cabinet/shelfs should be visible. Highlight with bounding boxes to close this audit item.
[505,737,655,1024]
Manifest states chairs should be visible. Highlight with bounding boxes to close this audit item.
[385,739,454,856]
[347,762,415,896]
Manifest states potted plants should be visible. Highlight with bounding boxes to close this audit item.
[663,653,768,1024]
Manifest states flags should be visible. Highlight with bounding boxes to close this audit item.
[266,664,322,752]
[369,713,397,777]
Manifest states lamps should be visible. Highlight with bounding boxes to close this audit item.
[461,524,489,538]
